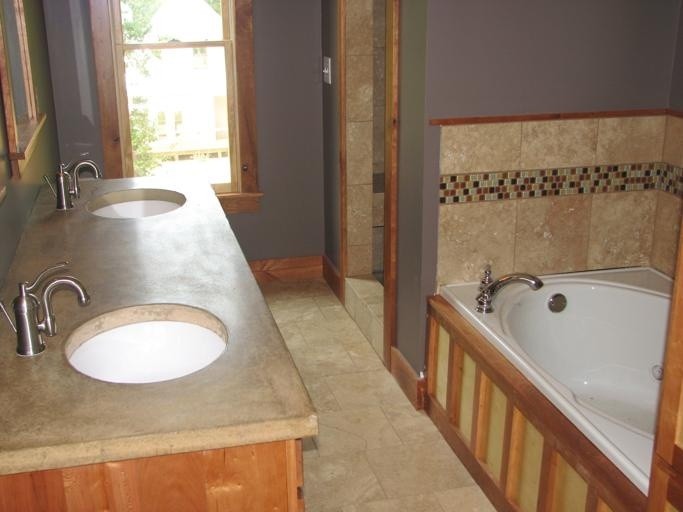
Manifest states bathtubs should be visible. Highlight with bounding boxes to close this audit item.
[439,267,677,495]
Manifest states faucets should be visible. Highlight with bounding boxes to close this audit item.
[477,266,543,311]
[13,263,91,354]
[56,151,100,211]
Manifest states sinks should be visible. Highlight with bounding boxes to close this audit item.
[83,187,185,218]
[66,304,229,386]
